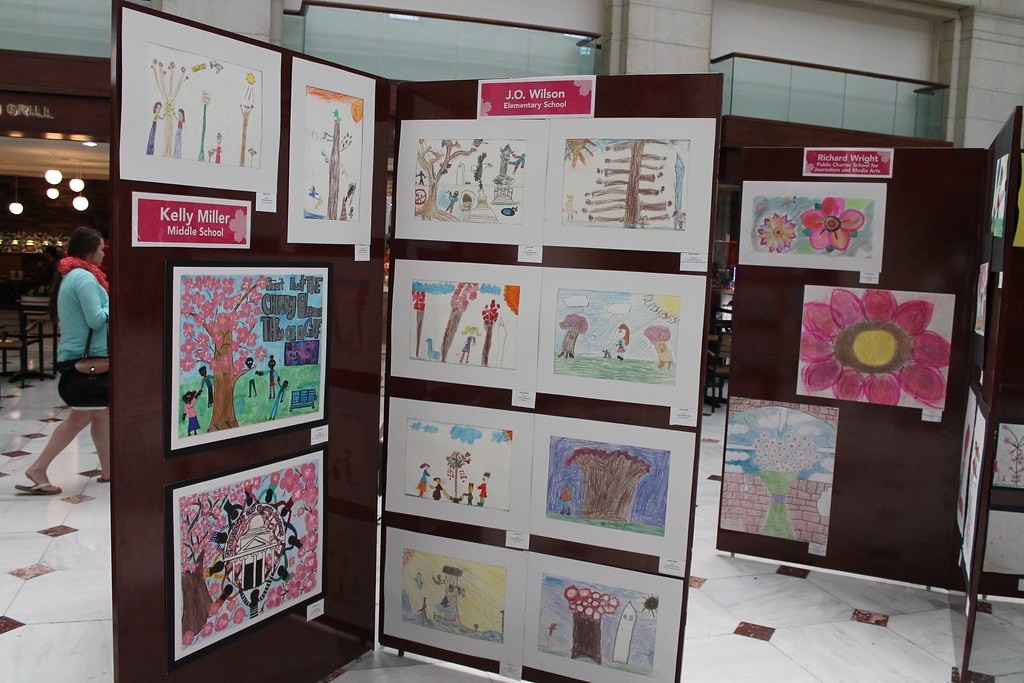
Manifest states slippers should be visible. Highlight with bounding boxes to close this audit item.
[96,477,110,482]
[15,483,58,495]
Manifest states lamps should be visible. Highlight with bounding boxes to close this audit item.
[8,132,92,214]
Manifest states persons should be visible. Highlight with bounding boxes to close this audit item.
[13,227,111,494]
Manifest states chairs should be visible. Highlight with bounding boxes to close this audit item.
[0,264,62,388]
[705,333,732,412]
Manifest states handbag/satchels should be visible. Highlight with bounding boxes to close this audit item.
[53,313,110,407]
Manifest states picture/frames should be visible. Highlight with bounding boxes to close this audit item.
[159,257,333,460]
[164,444,329,677]
